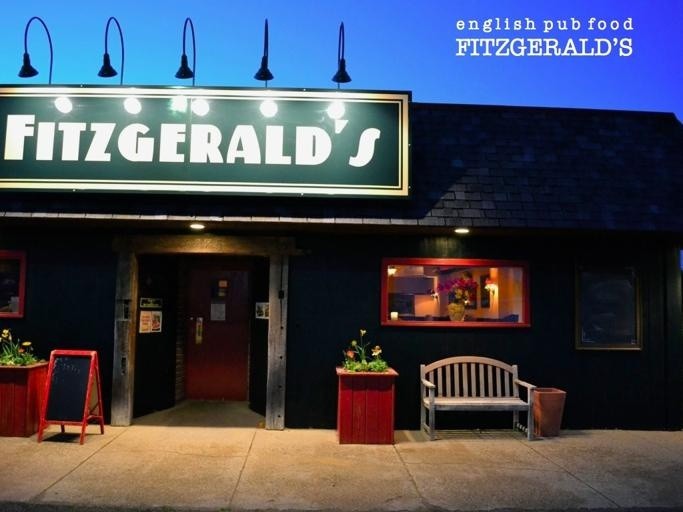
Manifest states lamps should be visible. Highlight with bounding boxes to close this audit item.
[17,17,52,85]
[1,249,26,319]
[332,22,352,89]
[97,18,125,86]
[174,19,197,86]
[254,18,274,88]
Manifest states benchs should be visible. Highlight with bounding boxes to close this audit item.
[418,355,537,440]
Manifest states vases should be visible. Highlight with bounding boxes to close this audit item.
[447,303,465,321]
[335,366,400,445]
[0,361,50,438]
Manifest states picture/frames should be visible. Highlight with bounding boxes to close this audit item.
[573,265,643,351]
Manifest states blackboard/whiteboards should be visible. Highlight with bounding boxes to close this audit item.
[39,350,94,425]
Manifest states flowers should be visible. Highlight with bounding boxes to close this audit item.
[436,278,479,304]
[342,330,388,372]
[0,327,39,365]
